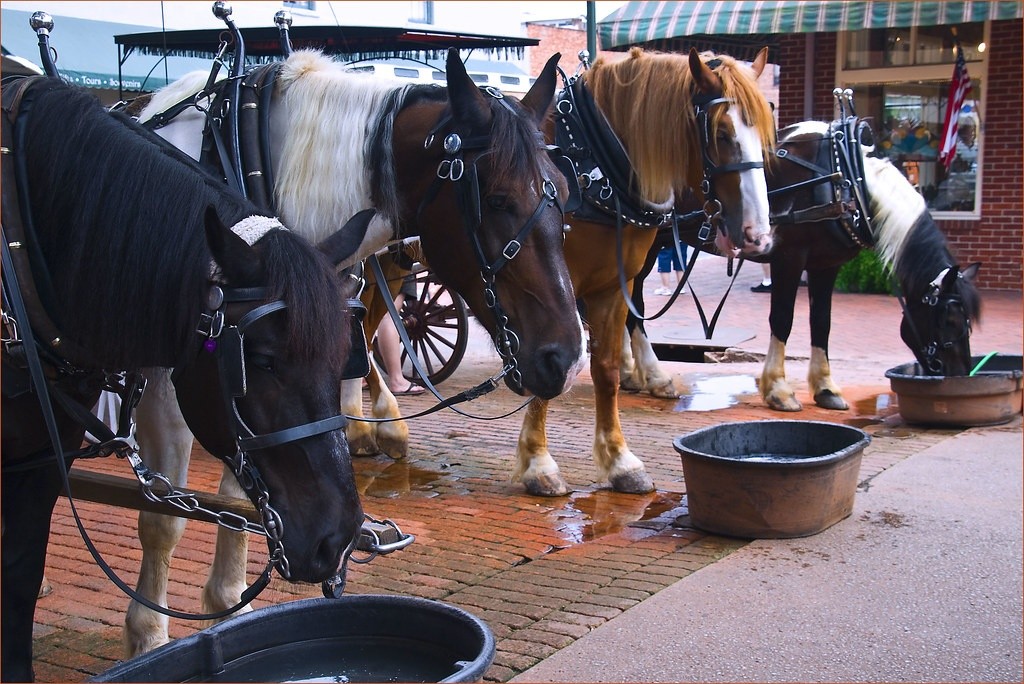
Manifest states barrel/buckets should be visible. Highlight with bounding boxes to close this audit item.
[884,352,1023,427]
[673,420,871,539]
[83,594,495,684]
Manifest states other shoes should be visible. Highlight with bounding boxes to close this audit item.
[798,280,806,286]
[751,283,773,293]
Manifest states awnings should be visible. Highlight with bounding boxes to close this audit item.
[596,0,1024,51]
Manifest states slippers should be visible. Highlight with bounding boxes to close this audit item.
[362,382,369,391]
[392,381,426,396]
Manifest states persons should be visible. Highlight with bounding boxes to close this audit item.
[361,273,427,396]
[750,263,808,294]
[654,242,688,295]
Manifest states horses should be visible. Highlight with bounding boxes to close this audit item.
[619,119,984,413]
[0,58,380,683]
[344,45,778,499]
[107,45,591,662]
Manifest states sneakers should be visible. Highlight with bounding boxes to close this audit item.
[680,290,686,294]
[654,287,671,296]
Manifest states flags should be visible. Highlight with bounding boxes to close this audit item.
[937,48,976,169]
